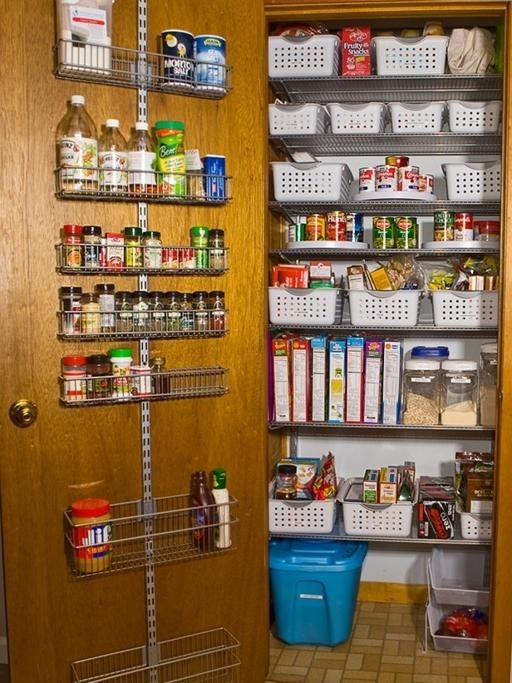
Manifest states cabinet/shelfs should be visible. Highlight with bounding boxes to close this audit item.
[268,68,507,549]
[419,546,490,655]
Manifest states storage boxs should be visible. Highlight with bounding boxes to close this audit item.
[267,536,368,646]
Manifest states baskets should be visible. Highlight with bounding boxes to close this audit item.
[442,161,501,200]
[268,477,419,537]
[268,98,503,135]
[372,35,449,76]
[269,287,498,326]
[269,162,352,201]
[267,35,340,78]
[455,503,492,539]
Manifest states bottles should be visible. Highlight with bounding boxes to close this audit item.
[129,121,156,193]
[275,464,298,502]
[150,355,170,394]
[192,470,214,549]
[480,342,497,428]
[88,356,112,396]
[443,358,478,428]
[97,118,131,197]
[211,468,232,547]
[60,283,225,333]
[400,344,449,427]
[65,223,225,272]
[62,356,88,400]
[57,93,101,196]
[110,347,134,395]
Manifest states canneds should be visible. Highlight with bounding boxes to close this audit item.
[358,155,434,196]
[70,498,113,574]
[61,282,225,336]
[62,224,225,271]
[62,347,152,401]
[160,29,194,90]
[372,216,419,249]
[193,35,227,93]
[434,209,500,241]
[288,210,364,243]
